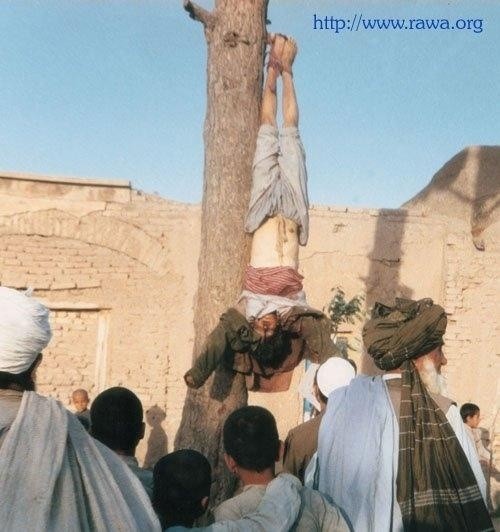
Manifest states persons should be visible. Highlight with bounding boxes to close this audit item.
[151,447,304,531]
[313,295,496,531]
[90,385,155,500]
[201,404,352,531]
[72,388,91,430]
[280,356,356,490]
[182,28,346,391]
[460,401,496,470]
[1,281,166,531]
